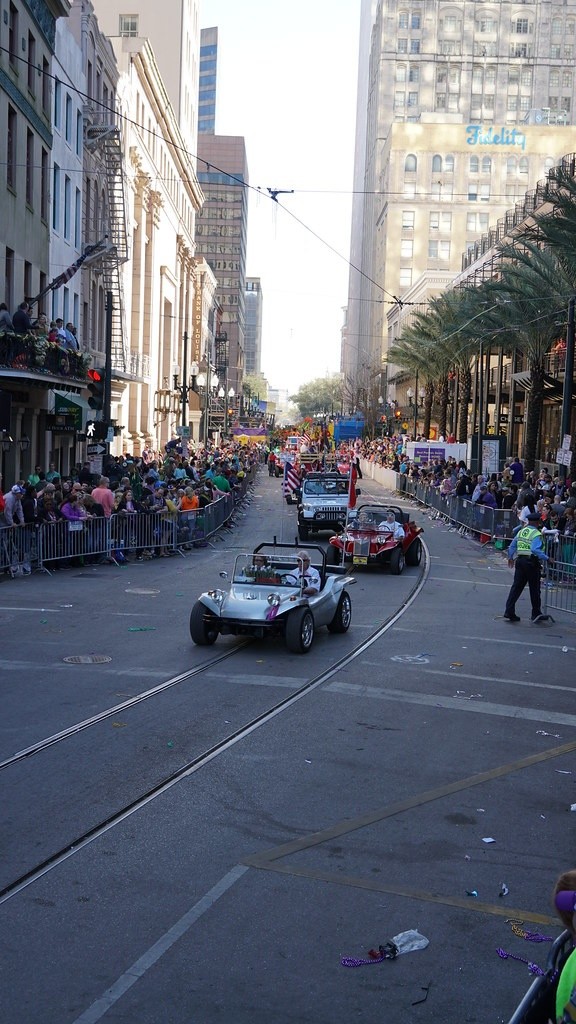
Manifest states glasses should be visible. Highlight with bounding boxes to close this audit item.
[386,514,394,517]
[35,469,40,471]
[544,503,550,508]
[297,559,309,562]
[254,558,263,563]
[74,489,83,492]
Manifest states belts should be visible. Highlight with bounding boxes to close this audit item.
[517,555,540,559]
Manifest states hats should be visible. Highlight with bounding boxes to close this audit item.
[479,486,488,491]
[520,481,530,489]
[526,512,542,521]
[12,485,26,494]
[545,475,552,481]
[543,468,549,474]
[563,507,574,516]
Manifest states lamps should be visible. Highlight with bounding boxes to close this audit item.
[17,432,31,453]
[0,432,13,452]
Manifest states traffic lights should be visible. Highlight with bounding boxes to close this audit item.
[87,368,105,410]
[85,420,107,439]
[395,408,402,425]
[381,415,388,429]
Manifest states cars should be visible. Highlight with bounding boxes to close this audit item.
[189,536,357,654]
[325,504,423,575]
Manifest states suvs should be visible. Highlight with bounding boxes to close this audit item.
[298,471,357,541]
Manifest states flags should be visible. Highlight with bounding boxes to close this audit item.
[51,248,93,291]
[299,432,312,444]
[281,462,301,497]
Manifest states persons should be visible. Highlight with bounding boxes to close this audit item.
[0,301,80,353]
[358,513,374,530]
[286,551,321,597]
[348,432,576,584]
[243,552,271,578]
[0,438,272,576]
[376,509,405,544]
[506,513,555,622]
[300,441,363,480]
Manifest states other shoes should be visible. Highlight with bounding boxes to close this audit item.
[531,610,549,621]
[7,521,234,574]
[504,610,521,621]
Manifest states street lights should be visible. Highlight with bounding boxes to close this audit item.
[169,330,199,427]
[218,379,235,436]
[406,369,427,441]
[378,385,392,436]
[195,352,220,448]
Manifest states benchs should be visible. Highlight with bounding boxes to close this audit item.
[271,569,293,584]
[353,512,409,528]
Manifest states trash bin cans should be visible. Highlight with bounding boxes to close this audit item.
[196,514,213,534]
